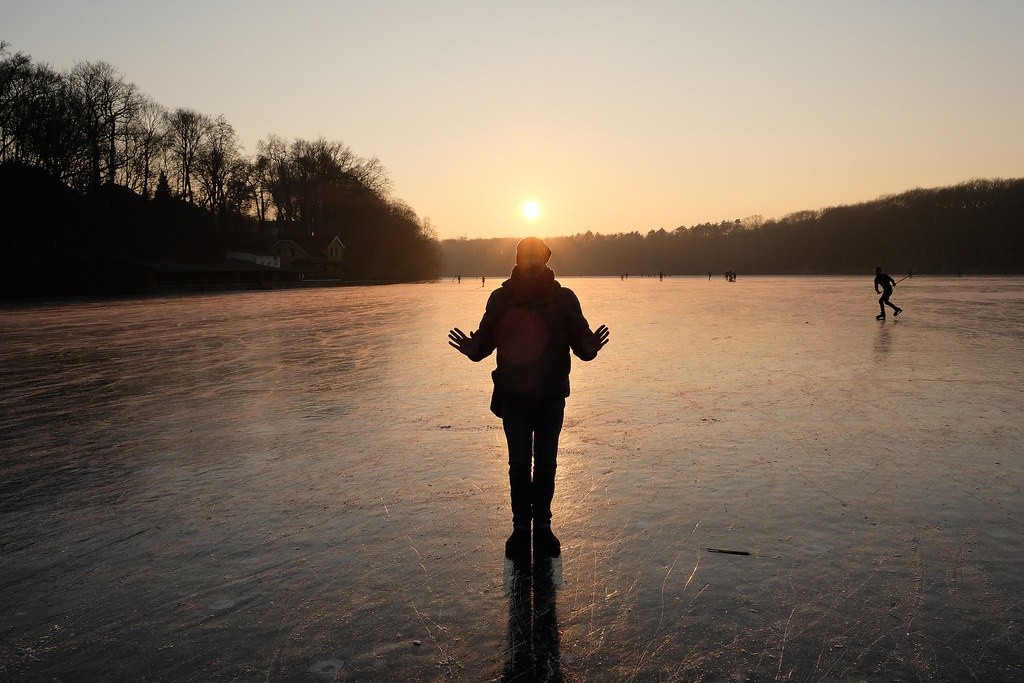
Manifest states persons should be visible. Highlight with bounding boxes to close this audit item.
[620,271,672,281]
[482,276,485,287]
[725,270,736,282]
[447,235,611,551]
[874,266,901,318]
[708,271,711,280]
[458,274,461,283]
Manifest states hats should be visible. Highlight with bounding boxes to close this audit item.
[515,237,552,262]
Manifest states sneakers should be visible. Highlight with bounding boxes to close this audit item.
[876,313,886,319]
[533,527,560,548]
[505,528,531,548]
[893,308,903,316]
